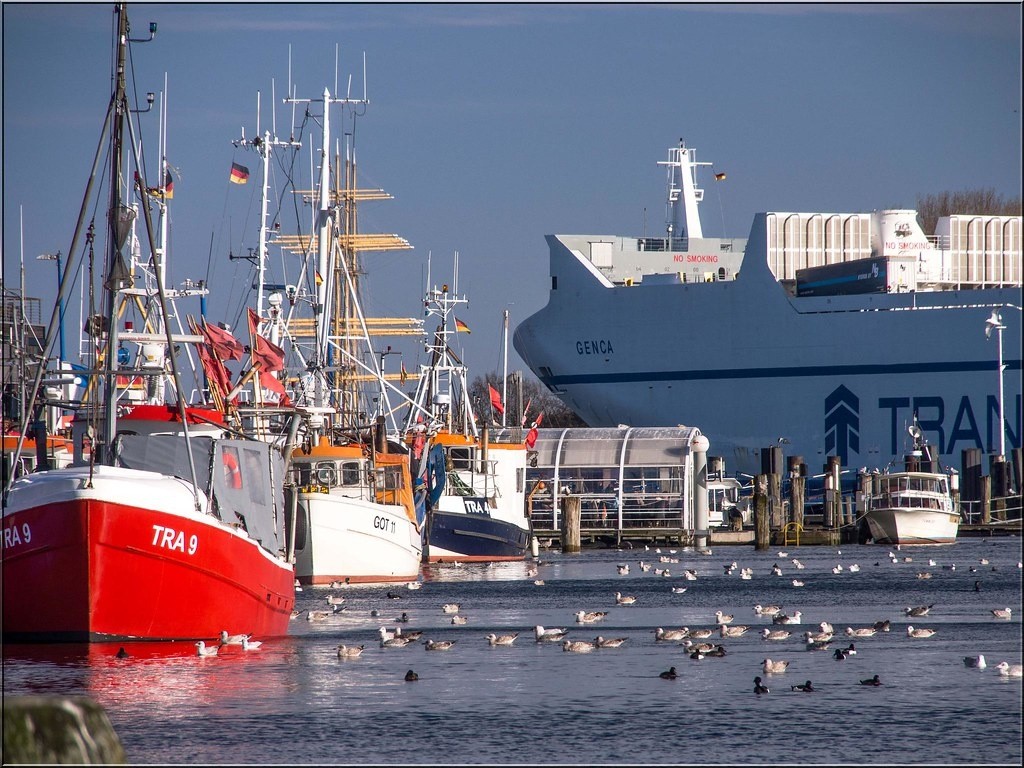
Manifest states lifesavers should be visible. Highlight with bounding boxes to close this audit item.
[222,453,242,489]
[348,444,367,449]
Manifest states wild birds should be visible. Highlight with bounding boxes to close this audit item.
[116,537,1022,695]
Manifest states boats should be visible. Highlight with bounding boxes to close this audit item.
[2,4,545,661]
[867,410,964,544]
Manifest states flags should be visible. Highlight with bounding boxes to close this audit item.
[399,304,542,448]
[135,168,174,200]
[313,272,324,288]
[192,310,297,418]
[229,163,248,184]
[716,172,725,181]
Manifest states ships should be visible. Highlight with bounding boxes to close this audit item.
[512,139,1022,523]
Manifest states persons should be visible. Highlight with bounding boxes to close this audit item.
[414,416,426,433]
